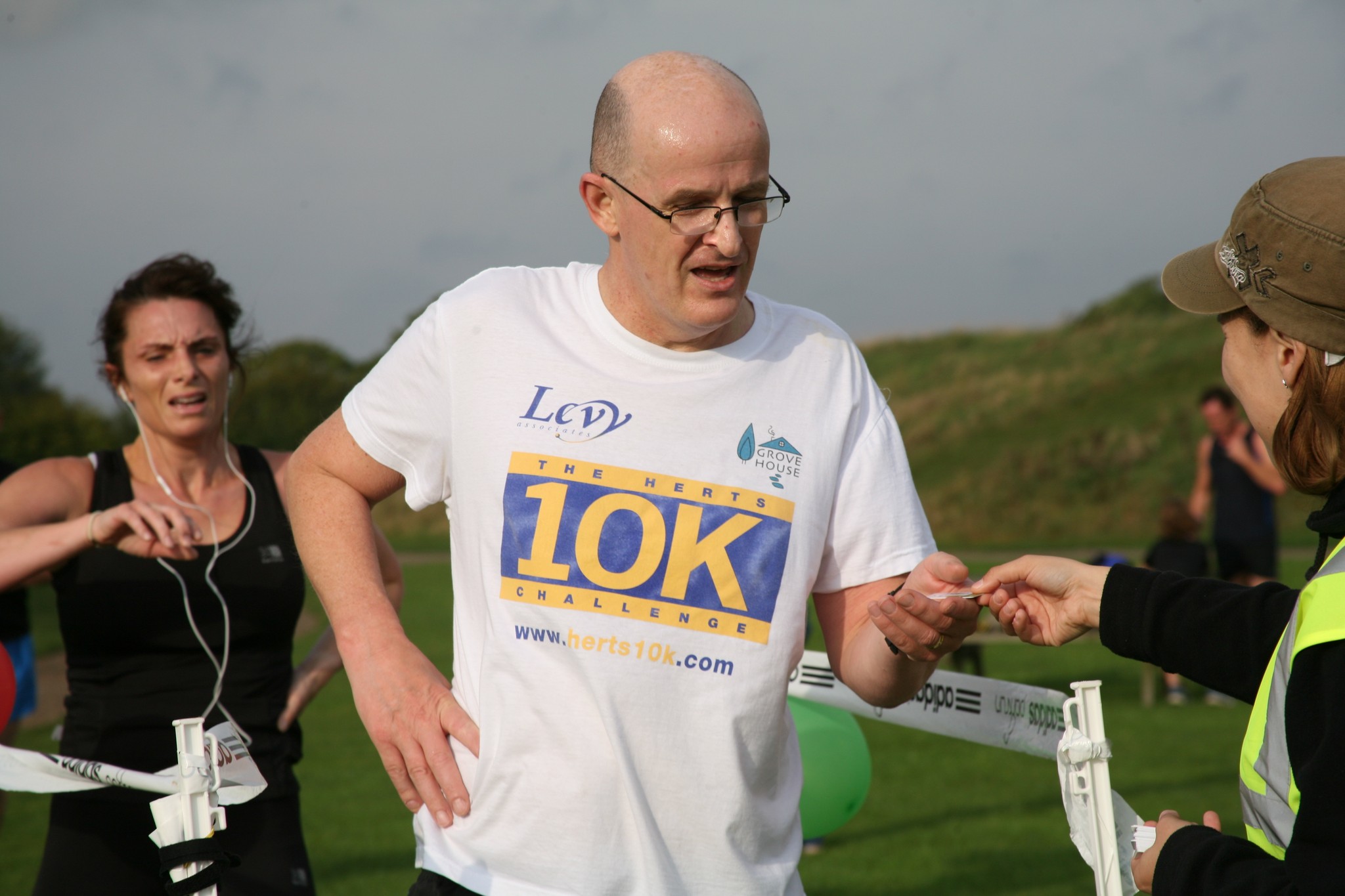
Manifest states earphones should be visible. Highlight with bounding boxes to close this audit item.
[227,371,234,389]
[117,383,129,402]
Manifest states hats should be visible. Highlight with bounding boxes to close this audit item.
[1161,155,1345,357]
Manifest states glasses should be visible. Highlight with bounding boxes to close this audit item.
[600,170,791,237]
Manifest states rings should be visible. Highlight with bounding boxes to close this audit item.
[925,632,945,650]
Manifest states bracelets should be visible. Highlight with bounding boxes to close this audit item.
[86,510,104,549]
[882,580,906,657]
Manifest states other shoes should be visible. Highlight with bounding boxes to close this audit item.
[1165,687,1187,707]
[1204,689,1234,708]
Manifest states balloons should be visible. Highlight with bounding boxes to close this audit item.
[784,696,872,843]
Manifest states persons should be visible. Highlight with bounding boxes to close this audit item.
[286,46,982,896]
[0,568,60,753]
[1136,495,1240,709]
[972,154,1344,896]
[1184,380,1287,589]
[0,248,405,896]
